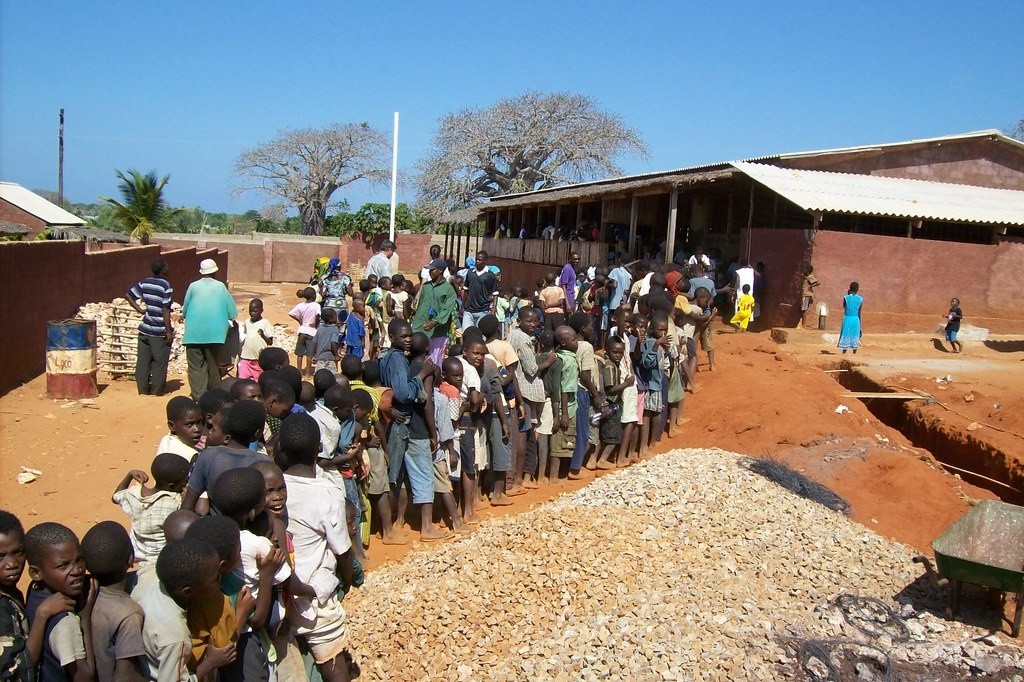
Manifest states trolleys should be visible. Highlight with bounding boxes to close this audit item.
[911,497,1024,640]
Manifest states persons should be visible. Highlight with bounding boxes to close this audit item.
[182,259,237,401]
[364,239,397,282]
[837,282,863,354]
[0,220,765,682]
[311,309,341,374]
[460,250,499,330]
[942,298,963,352]
[559,252,580,314]
[287,287,322,377]
[408,257,456,336]
[794,263,821,328]
[237,298,274,382]
[124,259,173,395]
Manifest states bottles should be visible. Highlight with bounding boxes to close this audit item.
[591,405,619,424]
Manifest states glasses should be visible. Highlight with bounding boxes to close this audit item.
[494,272,501,276]
[572,257,581,261]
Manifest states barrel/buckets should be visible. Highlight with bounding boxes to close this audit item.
[46,320,98,399]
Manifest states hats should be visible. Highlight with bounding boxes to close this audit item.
[423,258,446,271]
[466,256,476,267]
[199,258,219,275]
[487,266,500,274]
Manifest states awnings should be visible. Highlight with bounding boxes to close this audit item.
[732,156,1023,234]
[435,165,733,227]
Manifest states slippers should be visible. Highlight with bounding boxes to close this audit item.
[506,485,527,496]
[420,528,455,542]
